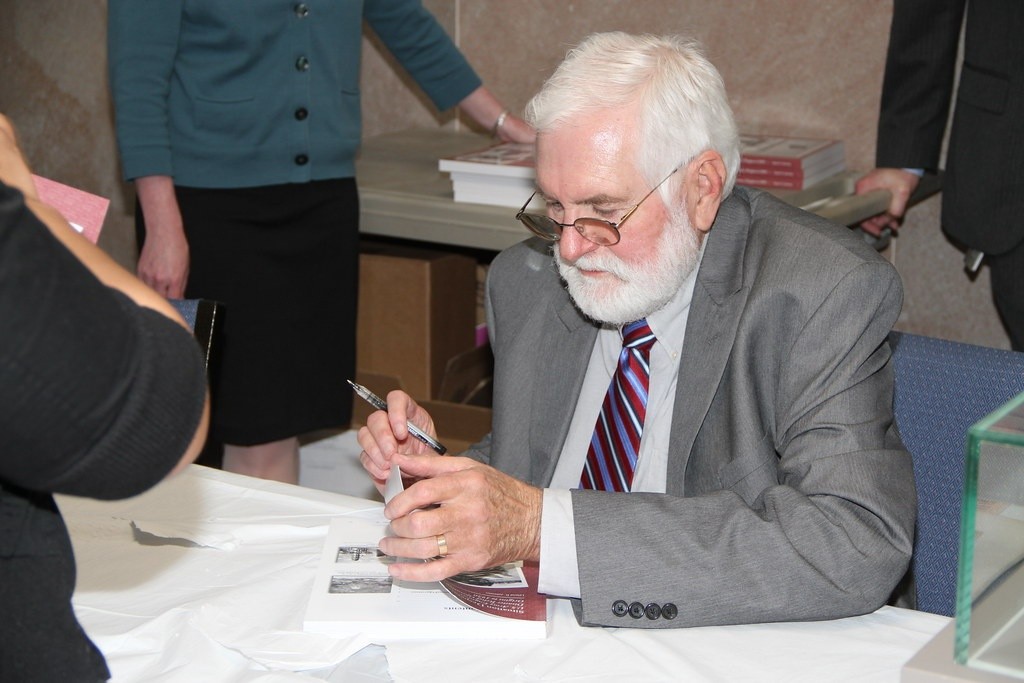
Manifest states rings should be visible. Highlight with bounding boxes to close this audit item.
[436,535,448,557]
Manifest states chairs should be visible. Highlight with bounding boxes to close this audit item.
[887,331,1024,617]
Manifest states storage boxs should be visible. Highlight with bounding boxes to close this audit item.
[351,230,496,510]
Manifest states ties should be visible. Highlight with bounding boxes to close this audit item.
[577,318,658,492]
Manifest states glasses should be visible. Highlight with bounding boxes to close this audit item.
[515,156,700,246]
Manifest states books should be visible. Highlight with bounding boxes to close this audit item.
[438,139,559,212]
[736,132,847,190]
[303,466,548,642]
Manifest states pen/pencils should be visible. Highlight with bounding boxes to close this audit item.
[345,377,448,458]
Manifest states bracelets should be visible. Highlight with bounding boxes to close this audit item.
[492,109,509,140]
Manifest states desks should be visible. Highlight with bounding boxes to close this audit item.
[354,126,860,252]
[51,460,956,683]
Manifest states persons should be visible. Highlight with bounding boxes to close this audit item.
[357,31,918,629]
[856,0,1024,352]
[0,108,210,683]
[109,0,536,488]
